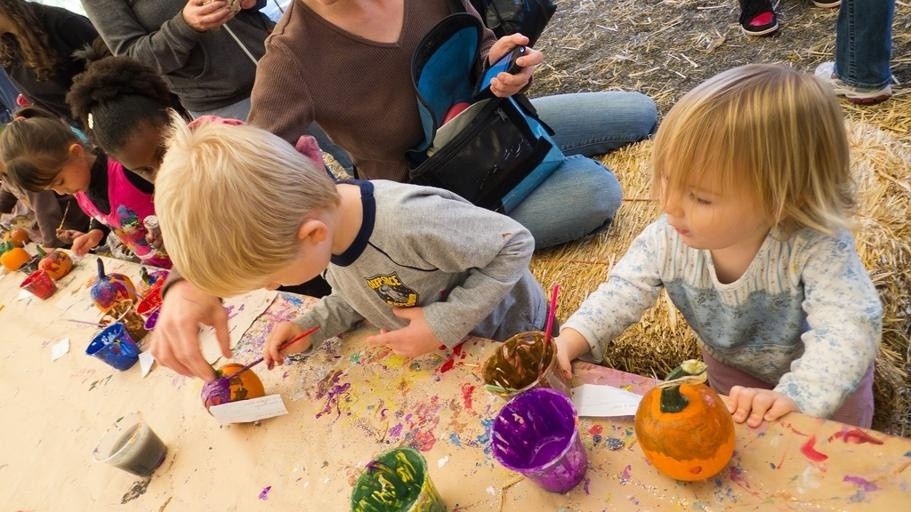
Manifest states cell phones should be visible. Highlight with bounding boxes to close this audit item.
[471,42,526,99]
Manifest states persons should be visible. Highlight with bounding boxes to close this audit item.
[1,1,354,270]
[151,0,660,385]
[551,62,883,433]
[814,1,895,105]
[152,113,560,372]
[738,1,842,35]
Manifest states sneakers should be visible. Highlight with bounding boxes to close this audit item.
[738,1,779,36]
[814,61,892,106]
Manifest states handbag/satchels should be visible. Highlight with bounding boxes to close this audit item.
[404,14,565,214]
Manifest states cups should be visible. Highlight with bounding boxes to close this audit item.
[19,254,57,300]
[478,331,587,492]
[85,288,168,371]
[89,410,168,477]
[343,448,451,511]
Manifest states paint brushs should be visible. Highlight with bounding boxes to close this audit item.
[484,384,519,392]
[68,319,108,327]
[538,284,559,376]
[56,200,71,237]
[119,280,154,307]
[227,324,321,380]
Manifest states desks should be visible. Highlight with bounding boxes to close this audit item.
[1,248,911,512]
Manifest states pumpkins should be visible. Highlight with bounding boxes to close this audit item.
[36,245,73,281]
[200,363,265,424]
[137,266,169,304]
[0,224,29,247]
[90,258,137,314]
[0,248,31,271]
[635,379,736,482]
